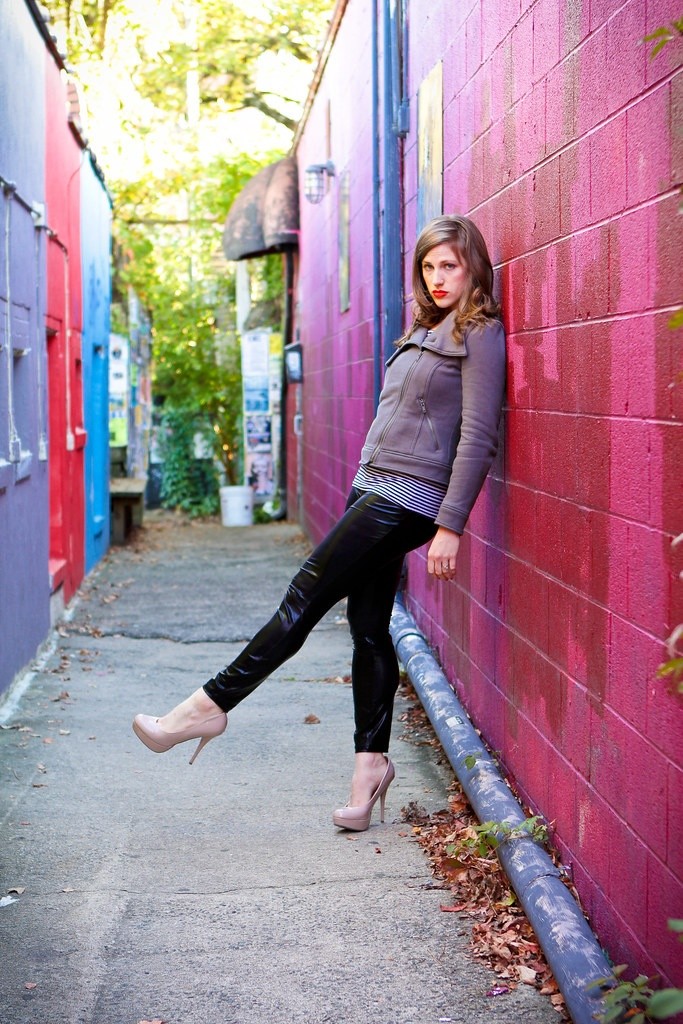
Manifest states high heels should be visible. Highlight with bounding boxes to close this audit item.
[132,711,228,764]
[332,755,396,830]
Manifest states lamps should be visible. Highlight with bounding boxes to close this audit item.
[304,161,335,204]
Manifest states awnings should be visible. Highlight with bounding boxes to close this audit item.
[218,155,297,262]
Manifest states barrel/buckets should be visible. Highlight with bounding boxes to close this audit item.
[219,485,254,527]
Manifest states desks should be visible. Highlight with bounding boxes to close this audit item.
[111,476,146,544]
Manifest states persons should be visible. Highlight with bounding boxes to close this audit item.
[248,456,273,497]
[132,213,506,831]
